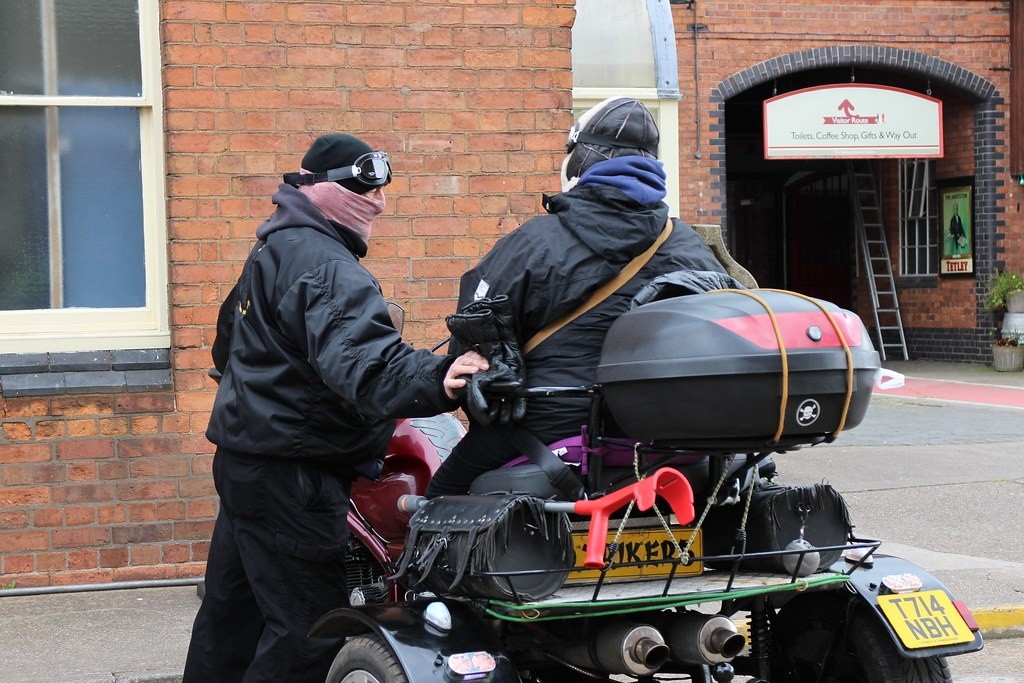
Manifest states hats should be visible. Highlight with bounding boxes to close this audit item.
[301,133,389,196]
[561,96,659,191]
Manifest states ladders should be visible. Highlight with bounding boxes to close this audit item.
[851,171,909,361]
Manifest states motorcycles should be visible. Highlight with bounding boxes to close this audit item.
[208,300,986,683]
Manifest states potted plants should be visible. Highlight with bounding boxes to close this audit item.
[983,270,1024,314]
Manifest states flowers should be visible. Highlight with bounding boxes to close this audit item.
[995,329,1024,348]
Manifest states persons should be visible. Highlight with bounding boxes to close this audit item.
[182,134,489,683]
[426,99,728,500]
[950,204,965,254]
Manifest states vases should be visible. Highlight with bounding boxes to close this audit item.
[992,345,1024,372]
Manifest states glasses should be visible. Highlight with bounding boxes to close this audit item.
[352,150,393,186]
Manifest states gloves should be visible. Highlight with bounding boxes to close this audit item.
[445,294,528,427]
